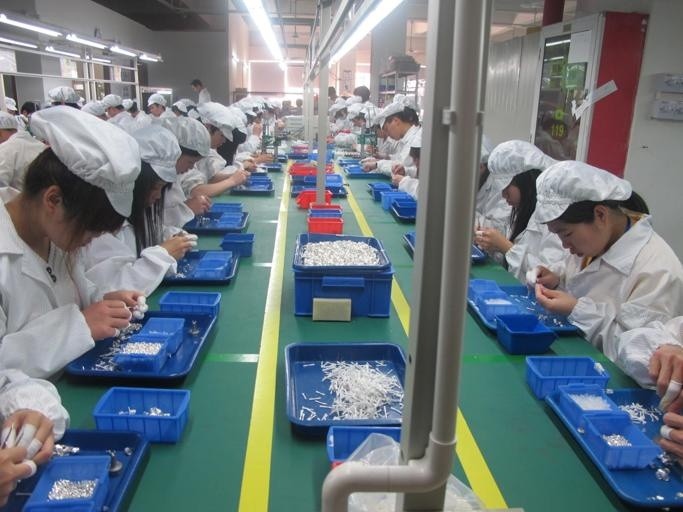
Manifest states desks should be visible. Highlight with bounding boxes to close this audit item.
[7,157,682,508]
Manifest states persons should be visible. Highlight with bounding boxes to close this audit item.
[2,79,301,512]
[526,160,683,355]
[328,86,423,199]
[616,316,683,468]
[473,139,556,271]
[476,145,511,217]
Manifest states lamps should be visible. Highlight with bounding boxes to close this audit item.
[0,12,164,67]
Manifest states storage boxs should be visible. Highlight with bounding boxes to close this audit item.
[292,232,393,320]
[307,202,344,234]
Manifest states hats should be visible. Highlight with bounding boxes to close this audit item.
[373,102,404,130]
[29,105,141,218]
[146,93,167,107]
[231,102,257,117]
[48,86,85,103]
[122,99,134,111]
[180,99,195,106]
[328,104,345,112]
[359,100,376,113]
[409,126,422,148]
[197,101,233,142]
[487,140,545,190]
[102,94,123,109]
[532,160,632,223]
[347,102,363,120]
[5,96,18,111]
[81,101,105,116]
[171,101,187,113]
[151,115,217,158]
[364,108,382,128]
[0,111,18,129]
[230,110,248,134]
[132,126,183,182]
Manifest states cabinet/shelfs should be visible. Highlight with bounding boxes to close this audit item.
[379,71,417,104]
